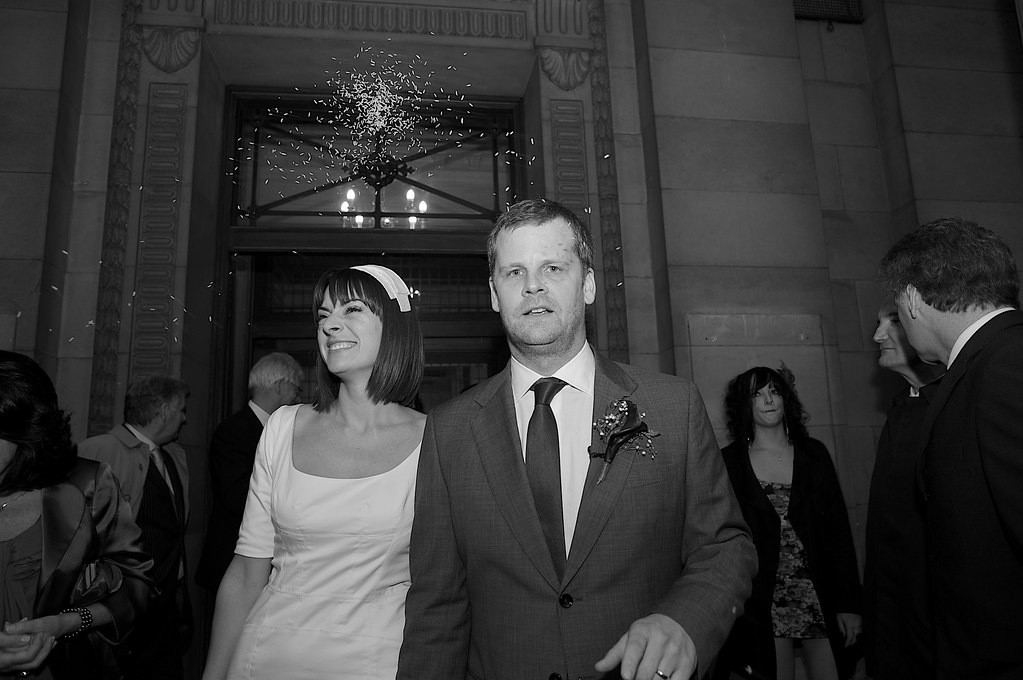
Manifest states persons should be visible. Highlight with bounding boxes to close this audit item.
[708,367,864,679]
[202,264,428,680]
[0,350,156,680]
[77,374,194,680]
[394,200,760,680]
[193,350,309,680]
[862,219,1023,680]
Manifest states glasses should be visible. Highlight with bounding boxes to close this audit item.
[289,380,304,396]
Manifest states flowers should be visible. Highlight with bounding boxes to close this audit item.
[592,394,660,487]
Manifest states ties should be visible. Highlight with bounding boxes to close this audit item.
[524,377,568,584]
[150,447,167,484]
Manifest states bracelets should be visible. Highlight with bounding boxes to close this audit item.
[58,607,93,640]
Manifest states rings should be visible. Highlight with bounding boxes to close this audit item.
[657,670,670,680]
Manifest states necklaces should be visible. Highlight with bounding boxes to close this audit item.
[0,491,27,511]
